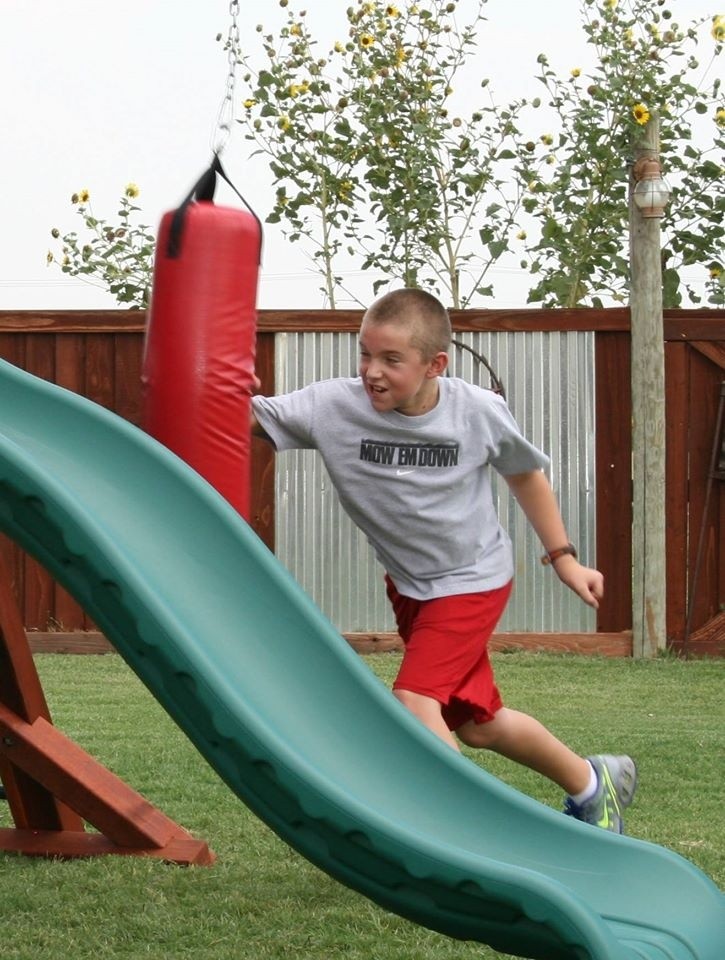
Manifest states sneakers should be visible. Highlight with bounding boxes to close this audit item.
[563,753,636,835]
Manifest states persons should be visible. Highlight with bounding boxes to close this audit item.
[248,287,637,836]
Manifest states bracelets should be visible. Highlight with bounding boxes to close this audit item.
[541,542,582,567]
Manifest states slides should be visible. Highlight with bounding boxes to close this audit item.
[0,353,725,960]
